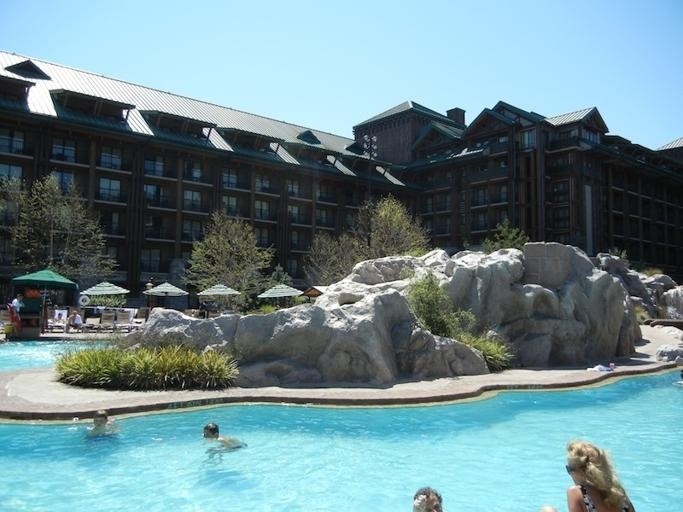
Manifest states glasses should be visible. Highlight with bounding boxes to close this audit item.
[565,465,575,474]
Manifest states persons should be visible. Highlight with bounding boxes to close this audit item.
[539,438,637,511]
[202,423,246,463]
[66,311,82,333]
[411,486,444,511]
[197,301,207,318]
[11,293,24,321]
[82,409,118,436]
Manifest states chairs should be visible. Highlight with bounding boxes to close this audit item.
[48,308,136,336]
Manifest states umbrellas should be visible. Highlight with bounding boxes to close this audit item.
[142,281,189,309]
[196,284,241,316]
[78,280,130,310]
[10,269,78,322]
[256,284,304,309]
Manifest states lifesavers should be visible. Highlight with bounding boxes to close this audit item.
[78,295,90,307]
